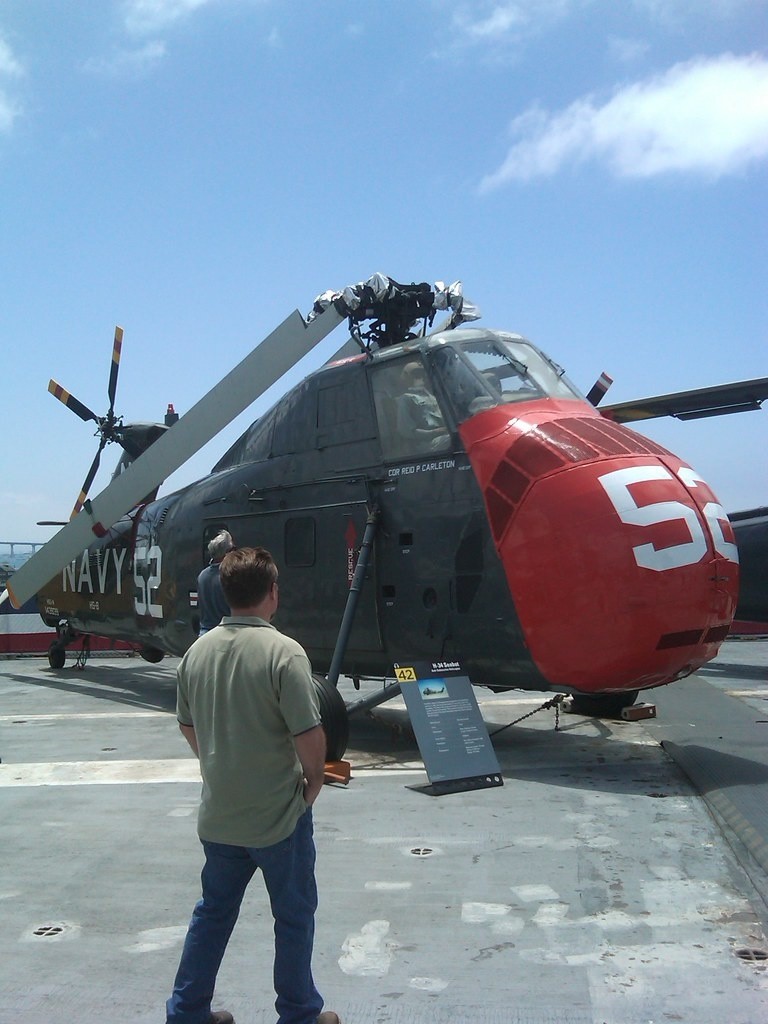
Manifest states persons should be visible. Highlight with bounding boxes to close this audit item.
[196,530,236,638]
[160,547,340,1023]
[395,362,503,441]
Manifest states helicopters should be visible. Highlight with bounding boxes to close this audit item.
[1,272,767,761]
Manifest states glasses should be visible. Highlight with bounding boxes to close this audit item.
[229,544,236,552]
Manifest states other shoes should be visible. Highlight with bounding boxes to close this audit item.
[317,1011,339,1024]
[211,1010,233,1024]
